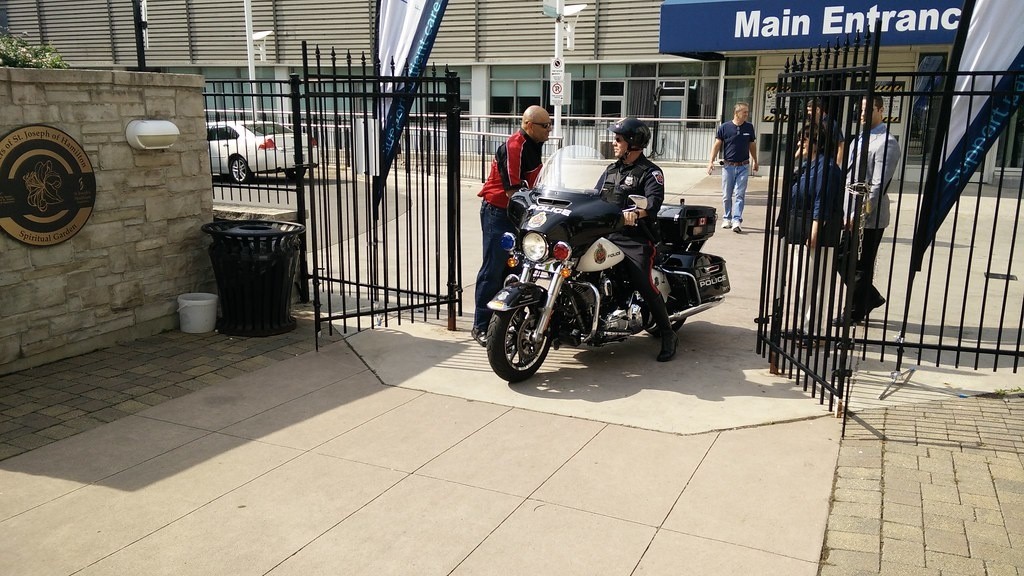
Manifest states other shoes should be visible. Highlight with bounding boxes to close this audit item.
[796,336,827,348]
[732,220,741,233]
[863,295,885,314]
[471,328,488,347]
[722,220,732,228]
[831,312,861,326]
[780,329,807,340]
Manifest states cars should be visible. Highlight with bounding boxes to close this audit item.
[207,120,318,184]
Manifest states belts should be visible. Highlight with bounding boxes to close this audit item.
[724,160,749,166]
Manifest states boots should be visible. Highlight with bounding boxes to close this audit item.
[648,292,679,361]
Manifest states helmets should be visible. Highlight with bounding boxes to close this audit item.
[608,118,651,148]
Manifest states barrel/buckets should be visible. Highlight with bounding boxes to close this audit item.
[176,293,219,334]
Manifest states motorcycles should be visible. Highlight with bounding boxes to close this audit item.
[487,144,731,382]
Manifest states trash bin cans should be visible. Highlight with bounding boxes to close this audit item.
[202,219,306,336]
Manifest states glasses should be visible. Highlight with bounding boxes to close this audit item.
[615,138,625,142]
[526,123,551,128]
[798,138,809,143]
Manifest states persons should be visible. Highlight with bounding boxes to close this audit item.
[594,118,680,362]
[774,98,845,348]
[832,94,901,326]
[707,103,759,233]
[472,106,552,347]
